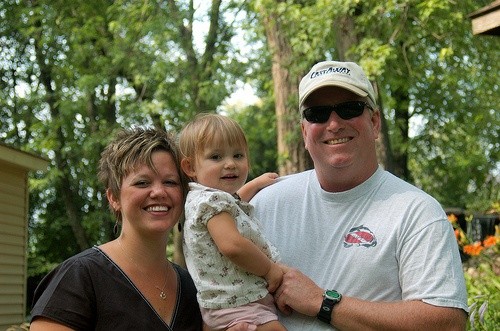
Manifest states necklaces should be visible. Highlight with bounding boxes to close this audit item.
[117,239,170,300]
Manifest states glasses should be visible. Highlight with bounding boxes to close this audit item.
[301,99,371,125]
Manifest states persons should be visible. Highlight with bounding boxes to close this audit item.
[204,60,469,331]
[29,125,203,331]
[175,113,285,331]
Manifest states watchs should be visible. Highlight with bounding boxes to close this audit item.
[318,290,342,325]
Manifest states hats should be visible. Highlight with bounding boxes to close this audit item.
[298,60,376,108]
